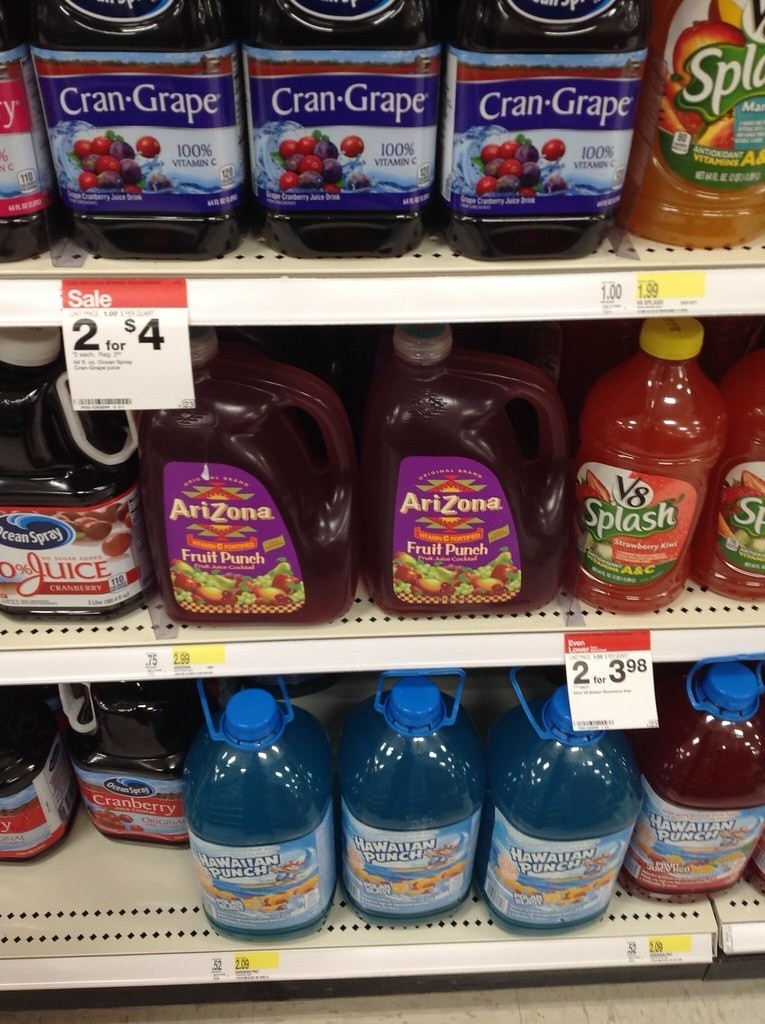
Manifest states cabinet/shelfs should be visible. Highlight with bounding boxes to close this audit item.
[0,245,763,998]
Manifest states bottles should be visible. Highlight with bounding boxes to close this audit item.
[0,1,764,938]
